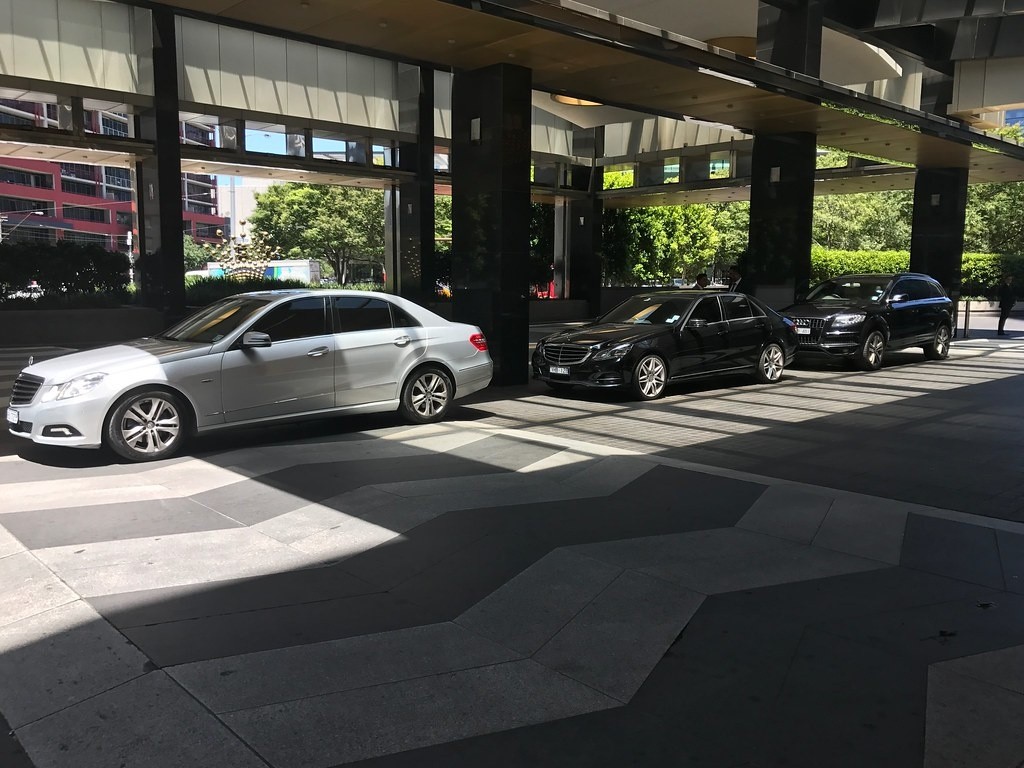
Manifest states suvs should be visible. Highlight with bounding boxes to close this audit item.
[776,272,956,371]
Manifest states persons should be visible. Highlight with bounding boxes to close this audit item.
[725,265,750,294]
[997,275,1019,334]
[693,273,709,290]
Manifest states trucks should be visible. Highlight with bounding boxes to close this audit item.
[184,261,321,285]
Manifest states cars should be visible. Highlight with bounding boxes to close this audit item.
[6,289,494,462]
[531,289,800,400]
